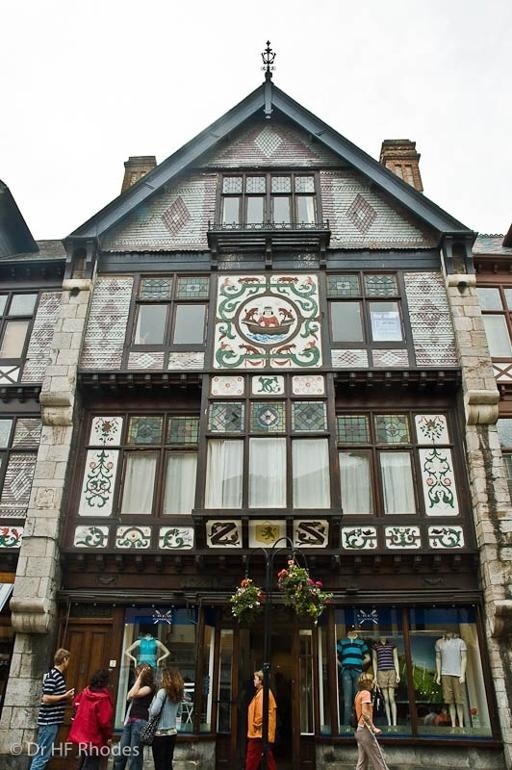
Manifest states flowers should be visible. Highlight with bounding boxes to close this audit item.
[230,578,264,624]
[277,557,334,625]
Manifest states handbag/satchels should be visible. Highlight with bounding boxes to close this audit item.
[139,714,160,745]
[69,702,80,721]
[349,711,358,729]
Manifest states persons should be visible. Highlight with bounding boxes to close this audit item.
[66,667,116,770]
[258,305,279,330]
[125,633,171,679]
[29,649,75,770]
[432,631,469,734]
[336,628,371,729]
[372,634,402,732]
[146,667,186,770]
[245,668,280,769]
[354,670,391,770]
[113,662,157,770]
